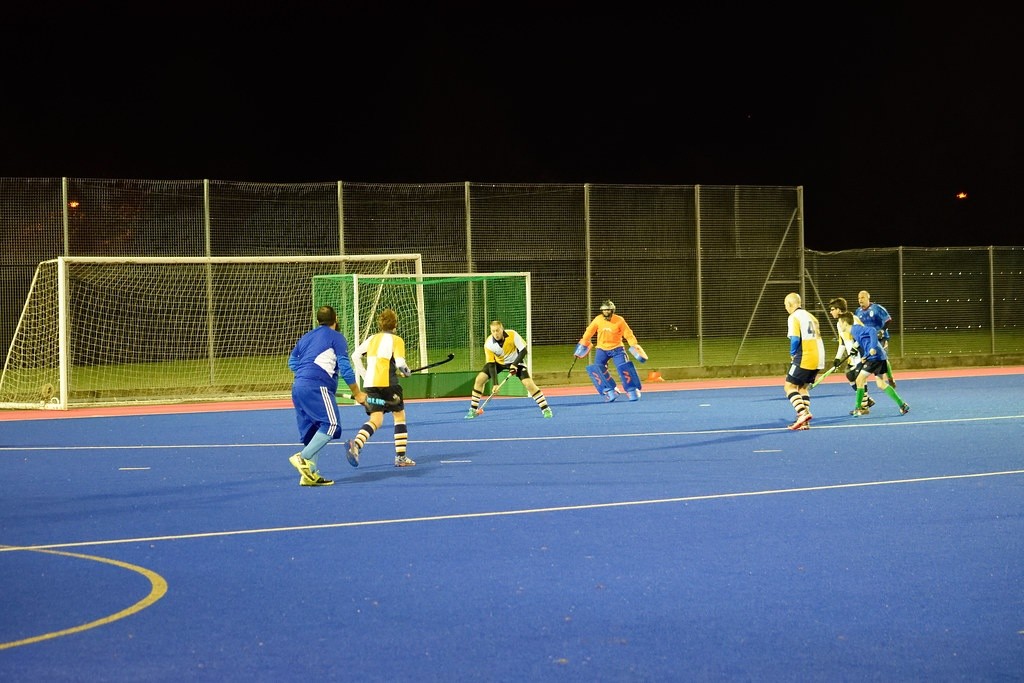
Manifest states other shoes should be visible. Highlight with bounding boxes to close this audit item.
[900,403,909,414]
[890,382,896,386]
[850,407,869,414]
[869,399,875,406]
[853,411,861,415]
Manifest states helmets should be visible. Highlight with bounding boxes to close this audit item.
[600,301,615,310]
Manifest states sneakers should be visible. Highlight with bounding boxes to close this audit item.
[395,456,416,467]
[344,440,359,467]
[792,410,812,429]
[300,469,334,485]
[464,409,479,419]
[544,410,552,419]
[788,422,810,430]
[289,452,314,481]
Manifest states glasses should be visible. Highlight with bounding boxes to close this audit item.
[829,309,836,312]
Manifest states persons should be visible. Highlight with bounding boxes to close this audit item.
[784,293,825,430]
[839,312,910,417]
[855,291,896,388]
[829,299,875,414]
[289,306,367,486]
[574,301,648,402]
[464,321,552,418]
[344,309,416,467]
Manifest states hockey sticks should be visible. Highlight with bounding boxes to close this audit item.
[567,356,578,384]
[846,354,871,374]
[410,353,454,373]
[334,393,401,406]
[474,373,512,417]
[807,353,850,390]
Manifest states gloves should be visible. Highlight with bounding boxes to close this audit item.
[877,331,882,339]
[861,357,867,363]
[834,359,841,372]
[850,348,857,357]
[870,348,876,355]
